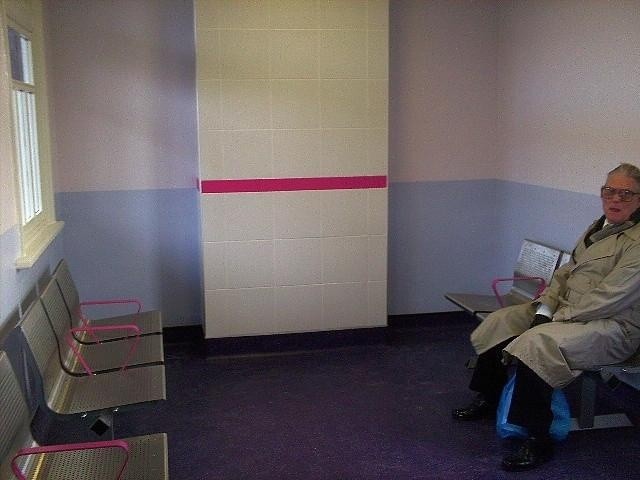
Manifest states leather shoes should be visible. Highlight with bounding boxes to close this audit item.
[452,397,498,423]
[500,436,552,472]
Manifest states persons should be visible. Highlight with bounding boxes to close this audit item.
[451,161,640,475]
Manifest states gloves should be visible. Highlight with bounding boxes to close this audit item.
[528,314,552,328]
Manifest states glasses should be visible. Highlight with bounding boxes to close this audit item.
[599,185,640,203]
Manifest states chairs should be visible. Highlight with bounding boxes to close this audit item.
[0,259,170,480]
[444,239,640,428]
